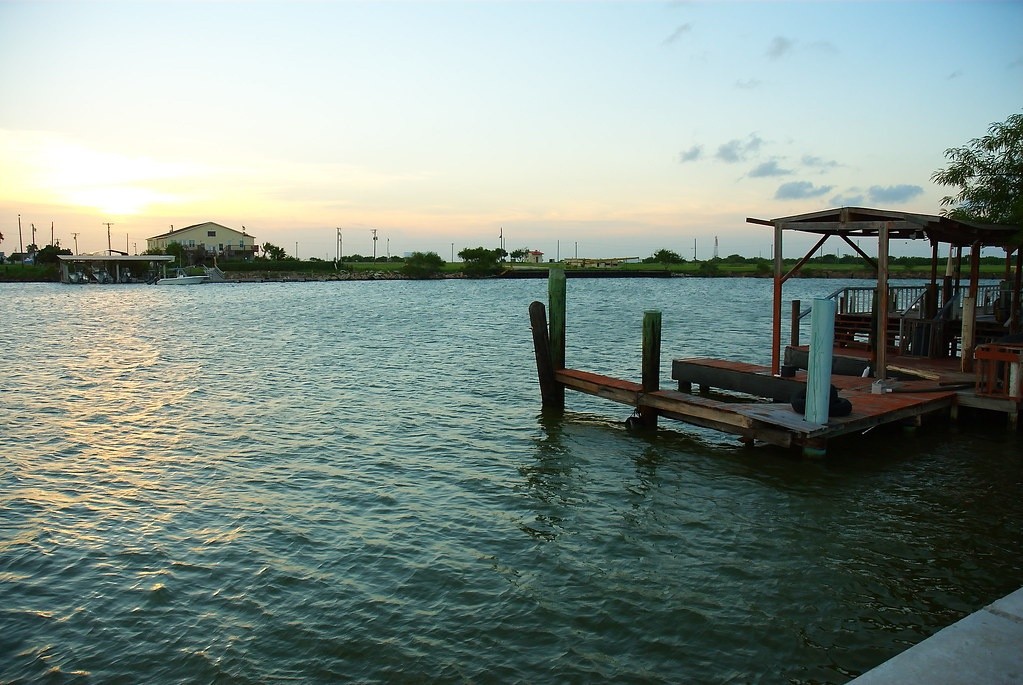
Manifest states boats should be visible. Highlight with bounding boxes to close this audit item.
[155,268,211,286]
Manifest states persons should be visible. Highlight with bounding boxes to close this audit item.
[123,268,132,279]
